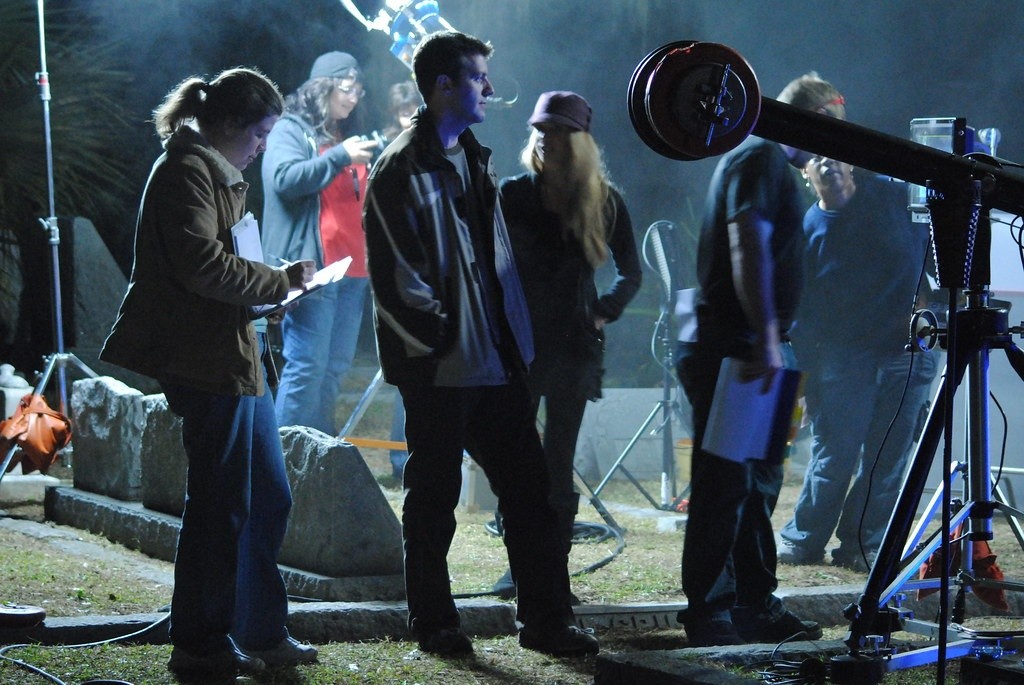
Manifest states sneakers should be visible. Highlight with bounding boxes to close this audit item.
[518,623,601,656]
[239,637,317,665]
[419,628,474,656]
[748,610,822,641]
[777,539,823,562]
[168,645,264,675]
[683,620,749,645]
[835,550,877,572]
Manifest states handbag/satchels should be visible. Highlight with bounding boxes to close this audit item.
[3,395,73,473]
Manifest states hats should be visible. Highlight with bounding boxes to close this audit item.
[527,90,593,129]
[309,52,365,82]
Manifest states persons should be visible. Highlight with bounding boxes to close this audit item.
[367,79,429,490]
[355,31,601,661]
[495,89,643,604]
[674,73,849,648]
[772,135,941,580]
[99,67,321,676]
[252,52,383,442]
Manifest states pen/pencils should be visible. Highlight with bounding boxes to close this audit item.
[268,253,293,266]
